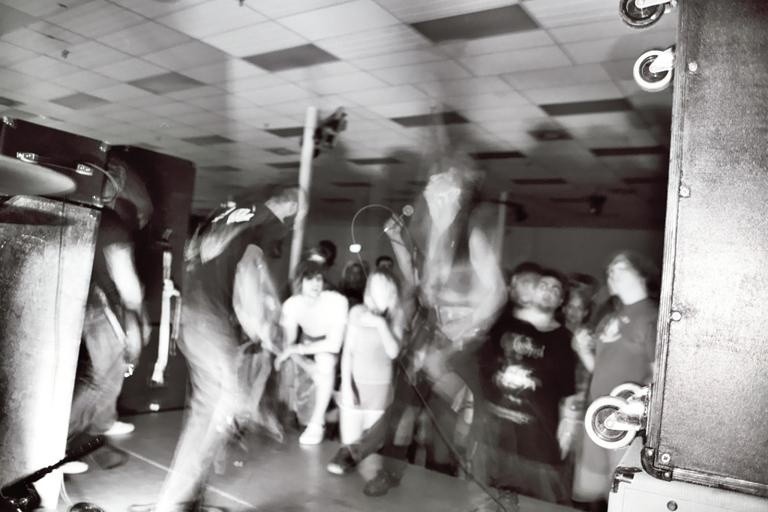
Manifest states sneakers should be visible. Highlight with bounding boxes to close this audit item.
[104,420,134,435]
[298,424,328,445]
[364,469,403,496]
[64,461,89,474]
[325,448,356,474]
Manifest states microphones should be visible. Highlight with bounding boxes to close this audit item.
[385,205,412,238]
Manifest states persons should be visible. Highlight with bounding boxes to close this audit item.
[62,150,657,511]
